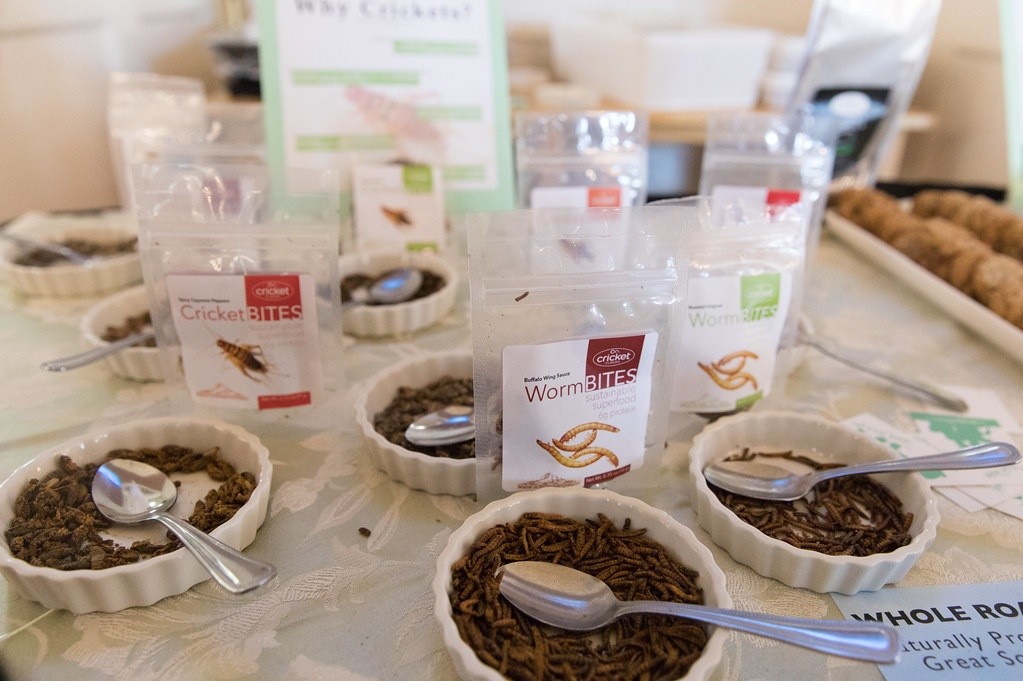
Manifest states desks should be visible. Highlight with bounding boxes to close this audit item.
[509,83,940,180]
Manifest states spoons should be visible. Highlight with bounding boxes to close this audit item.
[495,560,899,665]
[703,440,1023,501]
[342,266,422,310]
[404,405,475,446]
[92,459,278,595]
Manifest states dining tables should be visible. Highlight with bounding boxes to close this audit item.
[0,202,1023,681]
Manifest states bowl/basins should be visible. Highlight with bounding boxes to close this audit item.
[0,216,143,294]
[83,284,166,382]
[354,347,476,497]
[688,410,940,595]
[336,248,459,337]
[0,416,273,615]
[432,485,733,681]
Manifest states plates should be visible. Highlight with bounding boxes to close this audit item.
[823,195,1023,368]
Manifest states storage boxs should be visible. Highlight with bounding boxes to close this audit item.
[549,7,775,113]
